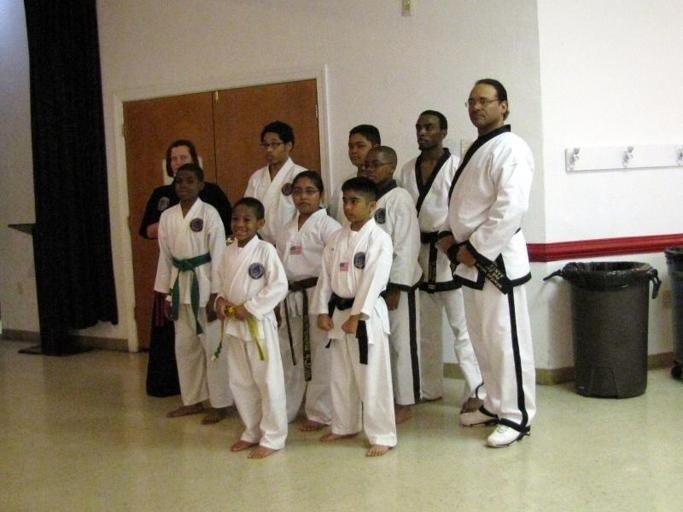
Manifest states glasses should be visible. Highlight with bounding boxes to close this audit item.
[465,99,498,108]
[260,143,280,149]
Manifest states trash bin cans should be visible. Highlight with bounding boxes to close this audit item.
[543,261,662,399]
[664,247,683,382]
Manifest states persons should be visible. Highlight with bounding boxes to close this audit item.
[141,118,342,422]
[308,175,397,457]
[440,81,535,451]
[211,196,288,459]
[395,105,485,413]
[325,121,425,424]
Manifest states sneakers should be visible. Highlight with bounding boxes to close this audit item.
[460,408,497,428]
[488,424,530,448]
